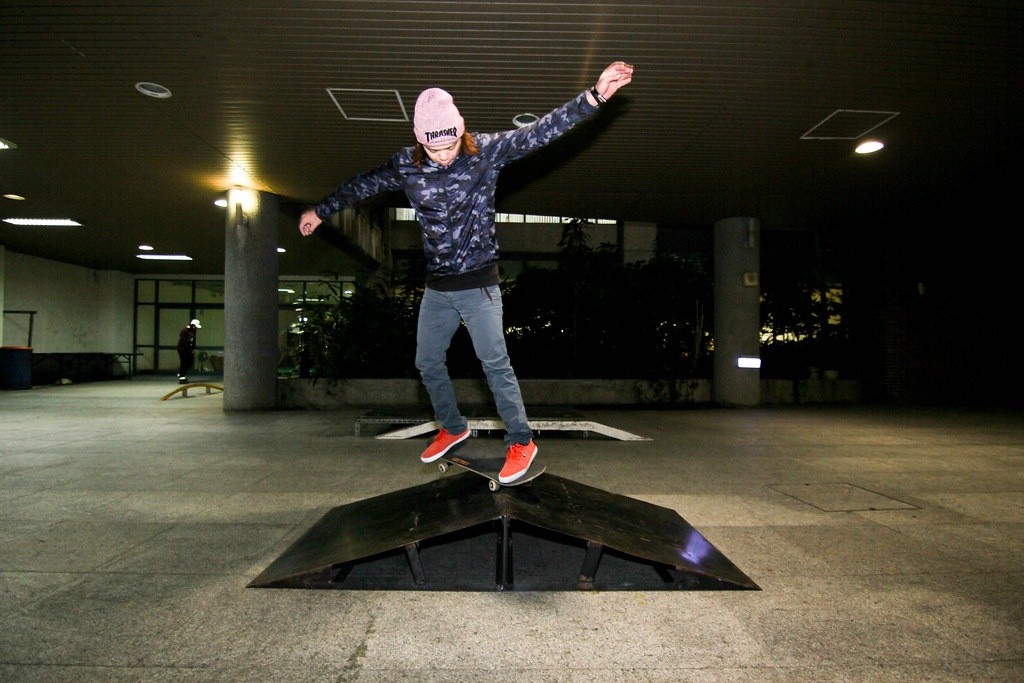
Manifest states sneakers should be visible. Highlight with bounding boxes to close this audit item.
[498,438,539,485]
[421,424,471,463]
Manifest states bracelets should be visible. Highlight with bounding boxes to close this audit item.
[590,85,607,106]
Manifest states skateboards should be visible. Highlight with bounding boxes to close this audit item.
[426,433,547,491]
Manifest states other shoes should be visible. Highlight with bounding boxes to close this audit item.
[178,374,185,380]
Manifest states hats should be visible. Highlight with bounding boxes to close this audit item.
[191,319,202,328]
[413,88,464,147]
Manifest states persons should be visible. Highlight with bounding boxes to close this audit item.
[177,318,202,384]
[298,61,634,484]
